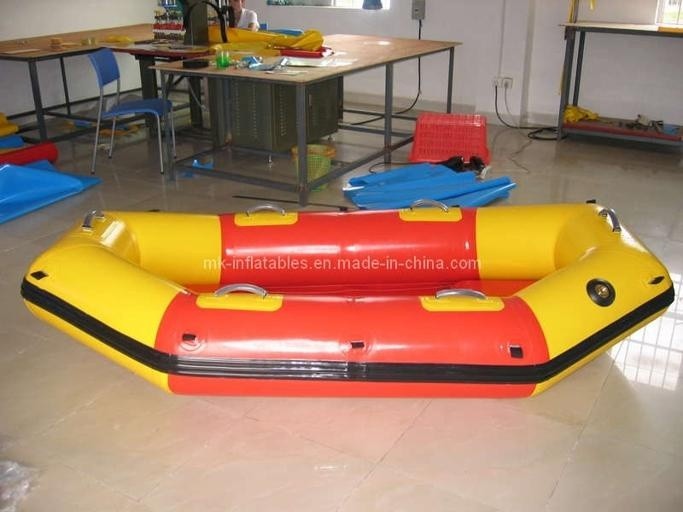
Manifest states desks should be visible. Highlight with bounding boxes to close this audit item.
[0,19,463,207]
[556,20,683,152]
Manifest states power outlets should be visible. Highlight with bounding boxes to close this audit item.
[489,76,513,90]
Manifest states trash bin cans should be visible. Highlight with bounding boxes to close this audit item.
[292,144,336,191]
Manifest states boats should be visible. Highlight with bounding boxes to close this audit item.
[20,198,675,399]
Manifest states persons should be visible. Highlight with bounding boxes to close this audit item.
[226,0,260,33]
